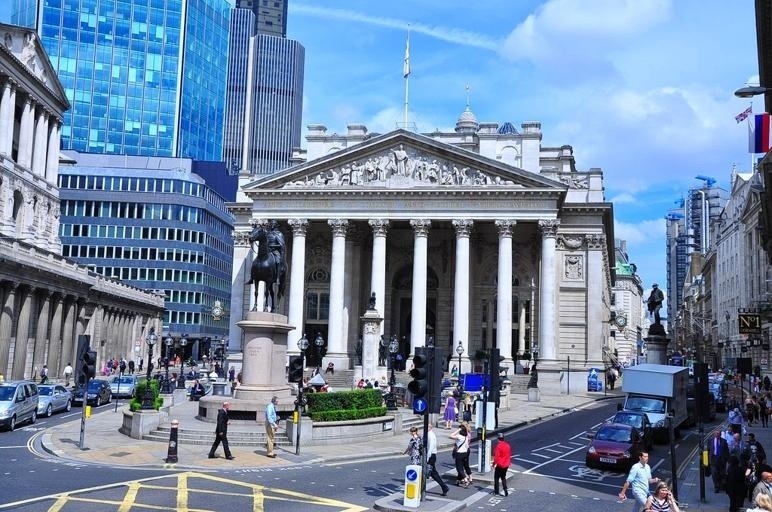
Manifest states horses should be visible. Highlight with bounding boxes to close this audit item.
[244,223,288,313]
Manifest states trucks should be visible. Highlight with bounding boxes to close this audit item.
[667,355,700,377]
[616,363,689,437]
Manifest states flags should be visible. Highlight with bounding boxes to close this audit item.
[403,36,411,79]
[748,113,772,153]
[734,107,752,124]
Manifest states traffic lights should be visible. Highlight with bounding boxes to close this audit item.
[290,357,303,381]
[407,346,434,397]
[83,351,98,374]
[491,348,506,390]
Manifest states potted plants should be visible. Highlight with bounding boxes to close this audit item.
[520,352,533,374]
[474,351,487,372]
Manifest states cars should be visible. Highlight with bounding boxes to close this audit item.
[74,379,112,405]
[38,384,75,418]
[587,423,637,468]
[612,411,652,447]
[109,374,138,398]
[706,372,727,400]
[139,373,174,384]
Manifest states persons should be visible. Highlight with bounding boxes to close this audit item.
[316,144,487,185]
[356,334,362,364]
[648,283,664,324]
[707,366,772,512]
[396,352,403,371]
[265,396,280,458]
[303,361,392,404]
[63,362,73,387]
[40,365,48,384]
[208,401,236,459]
[378,335,388,366]
[153,355,243,401]
[523,350,531,361]
[618,449,680,512]
[609,371,616,390]
[403,363,511,497]
[106,356,143,376]
[243,220,286,286]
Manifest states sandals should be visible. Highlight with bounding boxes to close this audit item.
[454,479,474,489]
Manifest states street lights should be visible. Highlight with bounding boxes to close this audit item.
[177,333,187,389]
[142,326,158,410]
[314,331,324,375]
[296,334,310,365]
[218,339,226,378]
[202,354,208,369]
[456,340,464,394]
[386,336,400,411]
[503,361,510,381]
[161,332,174,394]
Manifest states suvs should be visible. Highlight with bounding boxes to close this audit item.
[0,379,39,430]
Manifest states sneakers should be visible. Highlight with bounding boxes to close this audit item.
[267,454,277,458]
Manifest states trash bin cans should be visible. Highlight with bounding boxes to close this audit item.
[213,385,224,396]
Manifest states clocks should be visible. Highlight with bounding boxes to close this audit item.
[211,300,224,321]
[614,307,629,333]
